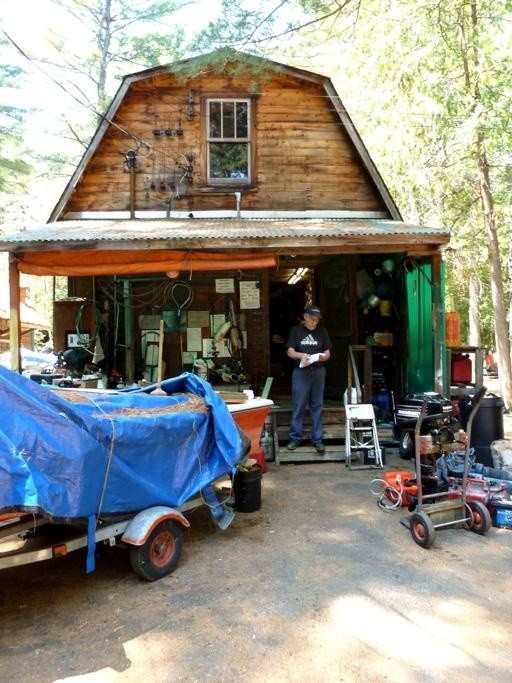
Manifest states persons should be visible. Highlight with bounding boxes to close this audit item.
[486,351,494,372]
[287,306,333,453]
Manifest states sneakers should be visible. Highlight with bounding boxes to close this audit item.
[288,441,299,450]
[315,442,324,452]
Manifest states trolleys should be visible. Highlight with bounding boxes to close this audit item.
[400,387,493,548]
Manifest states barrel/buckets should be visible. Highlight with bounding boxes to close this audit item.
[458,397,505,470]
[229,463,262,513]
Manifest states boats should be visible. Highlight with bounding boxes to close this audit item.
[0,388,274,580]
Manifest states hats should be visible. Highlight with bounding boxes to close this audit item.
[304,306,322,318]
